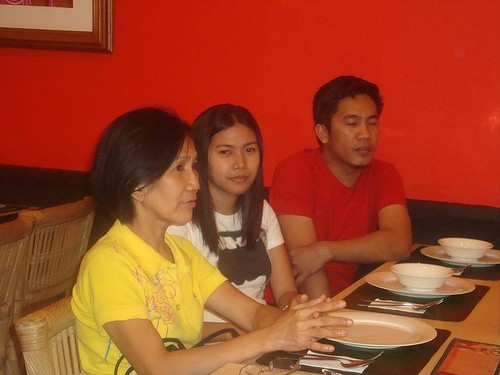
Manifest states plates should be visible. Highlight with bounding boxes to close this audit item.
[326,311,438,349]
[365,271,476,298]
[420,245,500,267]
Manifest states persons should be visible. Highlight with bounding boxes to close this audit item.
[71,107,353,375]
[263,74,412,303]
[164,104,298,346]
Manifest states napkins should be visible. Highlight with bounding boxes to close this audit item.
[447,266,464,276]
[368,298,430,314]
[298,349,369,373]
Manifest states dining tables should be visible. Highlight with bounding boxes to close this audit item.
[212,242,500,375]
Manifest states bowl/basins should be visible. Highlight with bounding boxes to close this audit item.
[438,238,493,260]
[390,263,454,291]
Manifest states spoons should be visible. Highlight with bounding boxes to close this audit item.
[357,298,444,311]
[277,350,384,368]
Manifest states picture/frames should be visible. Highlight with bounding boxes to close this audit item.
[0,0,112,54]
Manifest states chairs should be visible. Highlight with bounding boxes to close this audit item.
[0,196,97,375]
